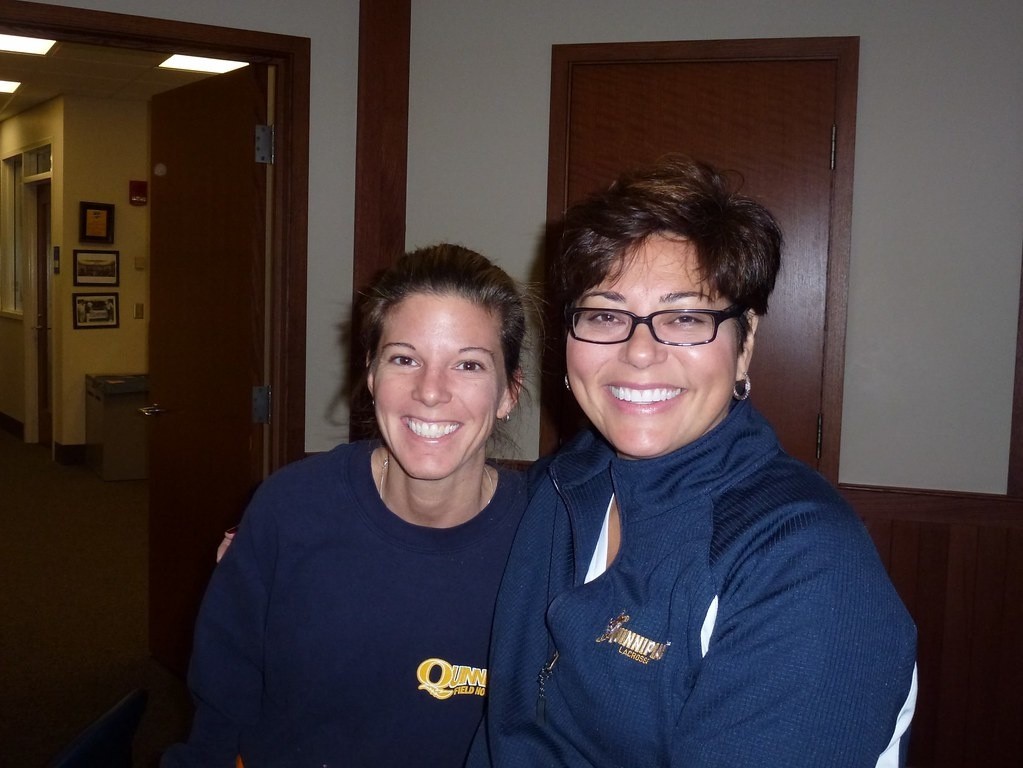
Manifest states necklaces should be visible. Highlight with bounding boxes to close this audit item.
[379,459,494,508]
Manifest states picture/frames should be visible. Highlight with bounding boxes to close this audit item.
[71,292,119,329]
[72,249,120,288]
[79,201,115,244]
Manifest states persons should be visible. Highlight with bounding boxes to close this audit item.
[163,244,531,768]
[461,152,920,767]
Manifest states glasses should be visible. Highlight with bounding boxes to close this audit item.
[563,298,746,348]
